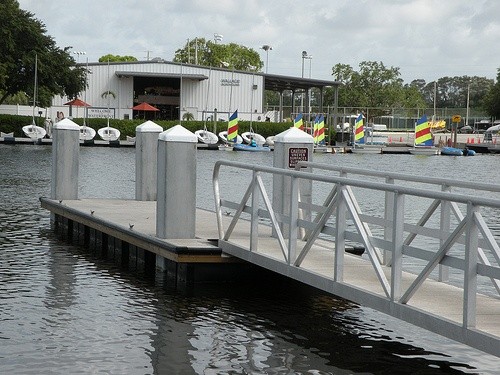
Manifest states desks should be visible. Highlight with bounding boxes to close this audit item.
[1,132,14,138]
[126,135,136,143]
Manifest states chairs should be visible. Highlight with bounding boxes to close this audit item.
[97,126,121,141]
[194,129,219,145]
[21,124,47,139]
[79,125,97,140]
[265,135,275,146]
[241,132,267,144]
[218,130,243,145]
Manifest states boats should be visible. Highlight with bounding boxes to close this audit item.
[232,142,271,152]
[265,133,276,150]
[441,146,477,156]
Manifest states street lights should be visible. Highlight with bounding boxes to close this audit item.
[301,50,308,78]
[262,44,270,74]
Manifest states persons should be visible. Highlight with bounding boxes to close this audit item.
[338,120,349,129]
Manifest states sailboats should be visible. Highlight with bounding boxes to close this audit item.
[218,64,244,145]
[240,68,267,147]
[79,56,96,142]
[97,57,121,142]
[20,54,47,139]
[313,113,442,156]
[194,62,220,145]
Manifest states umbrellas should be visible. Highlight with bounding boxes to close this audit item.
[63,98,92,117]
[130,102,159,120]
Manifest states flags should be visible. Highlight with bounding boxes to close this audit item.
[227,109,237,143]
[415,114,433,146]
[355,114,364,145]
[313,115,318,144]
[294,111,303,131]
[318,114,325,144]
